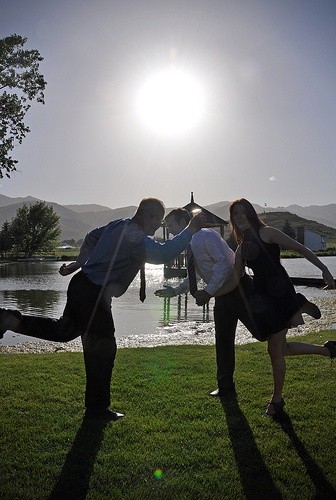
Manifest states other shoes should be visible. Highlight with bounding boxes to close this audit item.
[83,408,125,422]
[296,292,321,319]
[0,308,11,339]
[211,381,236,396]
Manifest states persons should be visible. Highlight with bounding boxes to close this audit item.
[153,209,322,397]
[0,198,205,421]
[195,198,336,420]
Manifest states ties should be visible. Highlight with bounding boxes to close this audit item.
[140,262,147,302]
[186,244,200,297]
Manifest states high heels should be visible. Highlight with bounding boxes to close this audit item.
[263,397,284,416]
[324,340,336,366]
